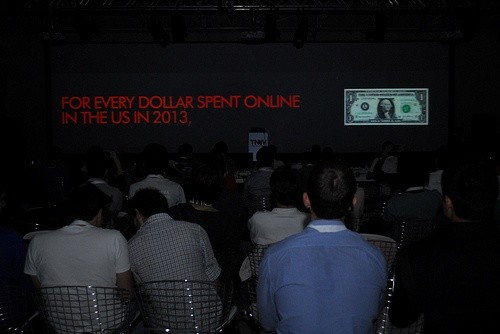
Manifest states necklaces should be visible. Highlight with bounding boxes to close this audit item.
[189,198,213,207]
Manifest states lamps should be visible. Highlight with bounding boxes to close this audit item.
[72,12,102,41]
[371,4,389,42]
[264,10,281,41]
[292,11,309,50]
[170,13,188,44]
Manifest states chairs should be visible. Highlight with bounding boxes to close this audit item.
[0,188,424,334]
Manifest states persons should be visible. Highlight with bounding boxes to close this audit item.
[231,167,310,290]
[0,196,31,324]
[390,159,500,334]
[125,187,225,334]
[255,162,388,334]
[31,138,467,236]
[22,186,135,334]
